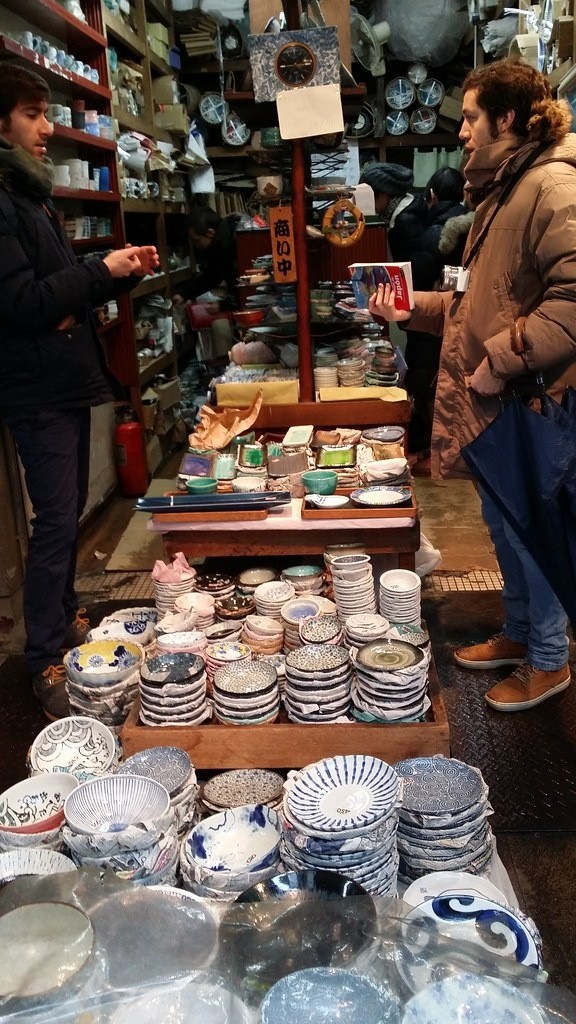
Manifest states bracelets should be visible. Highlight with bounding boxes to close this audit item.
[488,359,499,379]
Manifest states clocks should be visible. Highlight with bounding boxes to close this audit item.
[275,42,317,87]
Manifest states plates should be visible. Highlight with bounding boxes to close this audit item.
[308,492,350,508]
[351,486,413,505]
[85,566,554,1024]
[168,424,411,485]
[198,62,441,146]
[133,492,292,512]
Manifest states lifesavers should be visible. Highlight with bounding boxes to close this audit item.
[322,201,366,248]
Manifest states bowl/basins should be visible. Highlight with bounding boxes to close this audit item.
[256,176,283,196]
[301,472,337,496]
[187,478,218,495]
[226,256,392,392]
[0,536,422,1023]
[231,477,264,494]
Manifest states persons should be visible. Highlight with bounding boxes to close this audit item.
[1,61,160,721]
[360,163,476,474]
[170,207,241,313]
[369,58,576,711]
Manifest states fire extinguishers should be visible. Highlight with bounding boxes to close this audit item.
[115,409,151,498]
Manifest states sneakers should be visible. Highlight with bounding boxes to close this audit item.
[484,662,572,712]
[32,665,70,721]
[70,607,92,646]
[454,632,529,669]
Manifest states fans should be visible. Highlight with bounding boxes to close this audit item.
[349,14,392,76]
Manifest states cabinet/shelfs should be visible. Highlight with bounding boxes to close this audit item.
[102,0,198,480]
[180,25,484,194]
[209,84,415,464]
[0,0,145,595]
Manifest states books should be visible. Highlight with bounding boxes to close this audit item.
[187,301,231,331]
[348,262,415,309]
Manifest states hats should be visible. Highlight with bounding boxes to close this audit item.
[364,163,414,194]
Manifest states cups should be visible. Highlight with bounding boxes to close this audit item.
[7,25,111,189]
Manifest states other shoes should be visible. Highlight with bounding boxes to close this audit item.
[411,457,431,476]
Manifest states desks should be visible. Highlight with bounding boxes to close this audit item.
[237,222,388,318]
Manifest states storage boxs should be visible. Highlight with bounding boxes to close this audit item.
[146,23,169,63]
[152,75,190,135]
[152,492,268,522]
[216,364,299,406]
[142,374,190,475]
[436,85,465,133]
[122,621,450,769]
[301,486,417,520]
